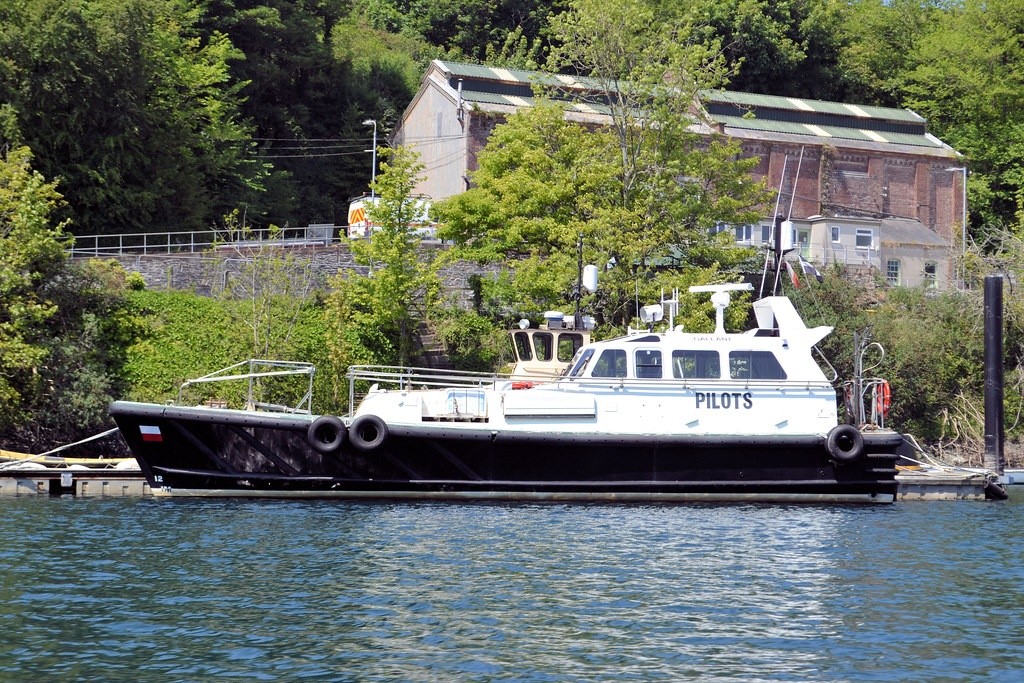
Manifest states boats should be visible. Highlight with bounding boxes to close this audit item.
[108,147,903,506]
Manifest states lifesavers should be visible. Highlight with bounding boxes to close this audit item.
[349,414,389,452]
[307,415,347,454]
[877,379,890,417]
[825,425,864,461]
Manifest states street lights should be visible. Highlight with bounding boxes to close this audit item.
[362,118,377,279]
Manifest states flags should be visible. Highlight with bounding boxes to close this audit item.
[787,263,800,288]
[799,255,823,283]
